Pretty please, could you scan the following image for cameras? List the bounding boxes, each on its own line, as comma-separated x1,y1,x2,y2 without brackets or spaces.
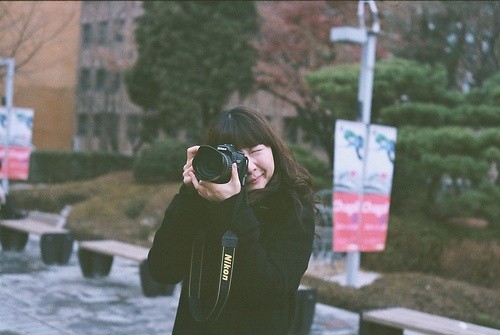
192,143,248,188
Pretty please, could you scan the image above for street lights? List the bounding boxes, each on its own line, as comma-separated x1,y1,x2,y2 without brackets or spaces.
331,1,380,287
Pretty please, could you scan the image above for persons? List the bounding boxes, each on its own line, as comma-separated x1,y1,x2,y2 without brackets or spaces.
142,110,319,334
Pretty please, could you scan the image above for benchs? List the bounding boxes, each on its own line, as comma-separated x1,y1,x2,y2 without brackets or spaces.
357,306,500,335
0,219,74,264
77,240,177,298
293,282,317,335
